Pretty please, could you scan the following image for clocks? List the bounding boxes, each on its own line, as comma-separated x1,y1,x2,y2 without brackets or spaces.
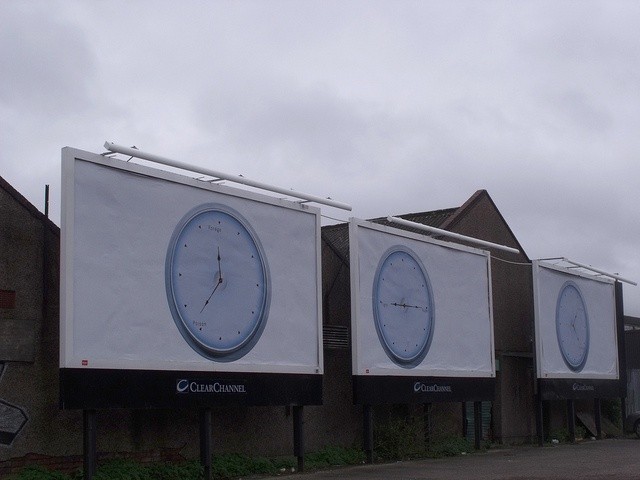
555,280,591,374
164,202,273,363
372,245,436,369
